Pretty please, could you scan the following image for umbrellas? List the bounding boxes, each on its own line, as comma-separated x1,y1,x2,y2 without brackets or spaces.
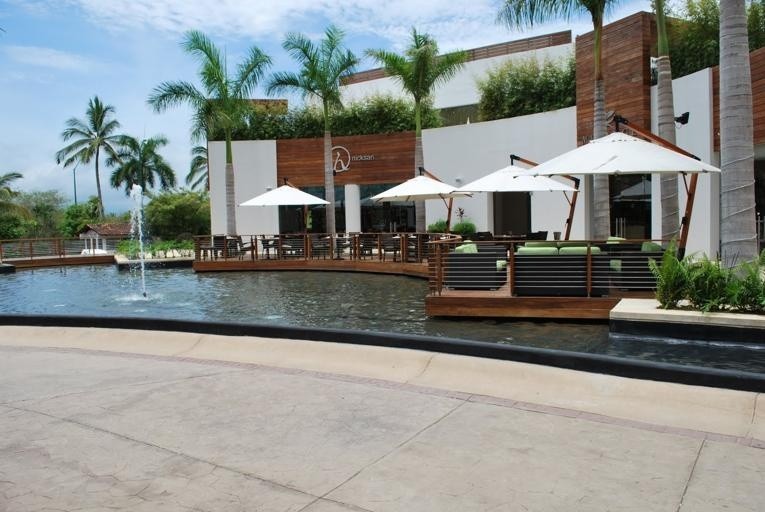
369,167,473,233
237,177,332,234
451,155,580,242
514,112,722,301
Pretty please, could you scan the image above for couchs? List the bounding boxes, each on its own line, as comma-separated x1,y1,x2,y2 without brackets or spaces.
429,225,669,299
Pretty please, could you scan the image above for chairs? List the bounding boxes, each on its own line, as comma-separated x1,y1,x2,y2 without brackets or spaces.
198,233,430,264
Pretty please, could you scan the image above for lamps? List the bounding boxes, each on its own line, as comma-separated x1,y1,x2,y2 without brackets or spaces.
674,110,690,125
